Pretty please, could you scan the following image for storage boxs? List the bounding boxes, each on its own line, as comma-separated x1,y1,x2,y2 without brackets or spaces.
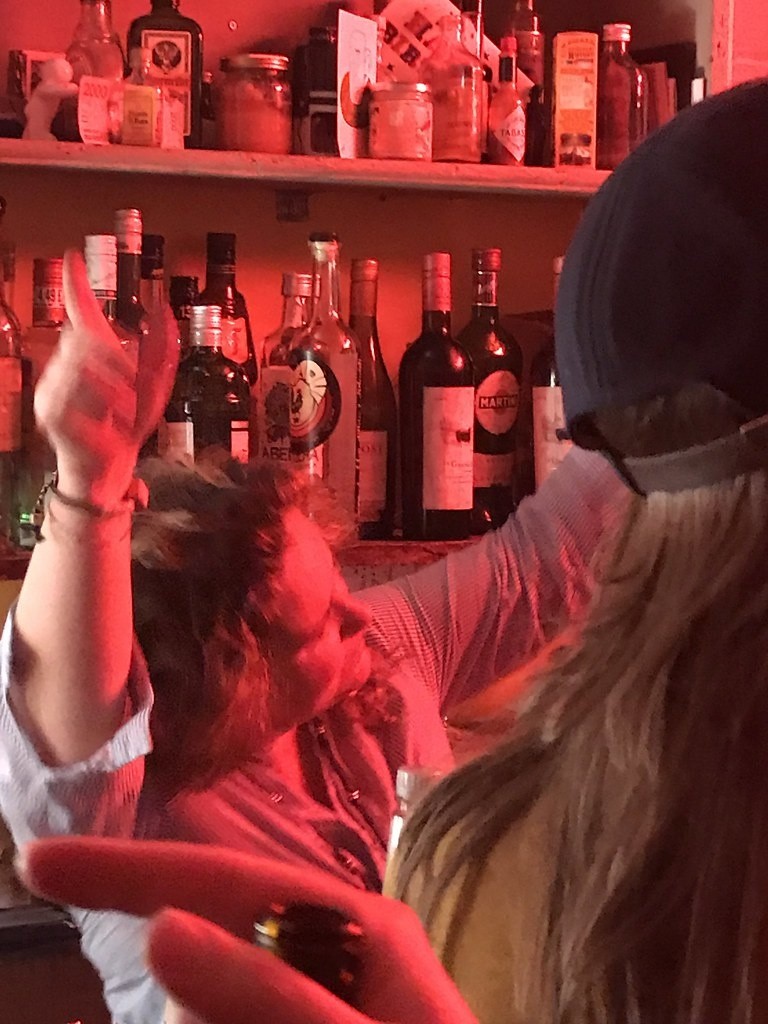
554,31,598,167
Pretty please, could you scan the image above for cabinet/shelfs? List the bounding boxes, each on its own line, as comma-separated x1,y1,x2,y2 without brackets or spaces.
0,1,734,588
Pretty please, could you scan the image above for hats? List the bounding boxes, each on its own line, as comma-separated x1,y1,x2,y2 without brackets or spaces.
554,75,768,493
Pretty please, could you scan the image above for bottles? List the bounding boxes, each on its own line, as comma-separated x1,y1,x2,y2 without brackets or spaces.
490,0,545,166
533,255,576,494
285,233,347,508
63,0,132,145
597,23,650,170
251,900,365,1010
204,26,336,156
167,232,258,468
261,273,330,483
121,47,163,145
381,764,433,893
454,248,524,536
125,0,203,147
82,208,165,459
398,250,474,540
0,242,70,548
422,0,492,163
348,257,397,541
368,82,432,160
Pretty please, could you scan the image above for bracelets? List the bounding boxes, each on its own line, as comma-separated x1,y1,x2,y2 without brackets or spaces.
19,477,149,543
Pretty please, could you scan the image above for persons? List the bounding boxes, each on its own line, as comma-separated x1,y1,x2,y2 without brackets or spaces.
16,837,475,1024
382,76,768,1024
0,246,626,1024
